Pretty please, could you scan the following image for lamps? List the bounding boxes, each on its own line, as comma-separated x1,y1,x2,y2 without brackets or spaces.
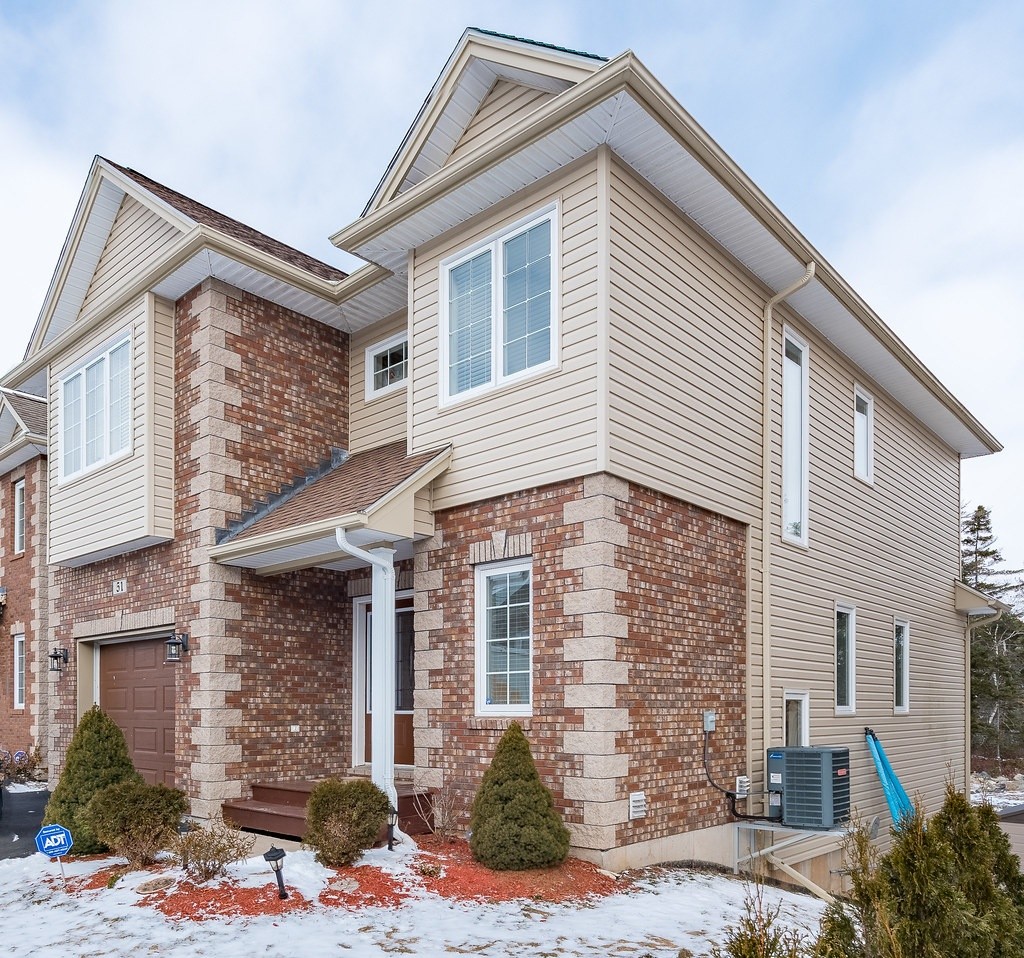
263,842,289,900
178,818,203,870
164,632,189,662
48,647,68,672
386,805,399,851
